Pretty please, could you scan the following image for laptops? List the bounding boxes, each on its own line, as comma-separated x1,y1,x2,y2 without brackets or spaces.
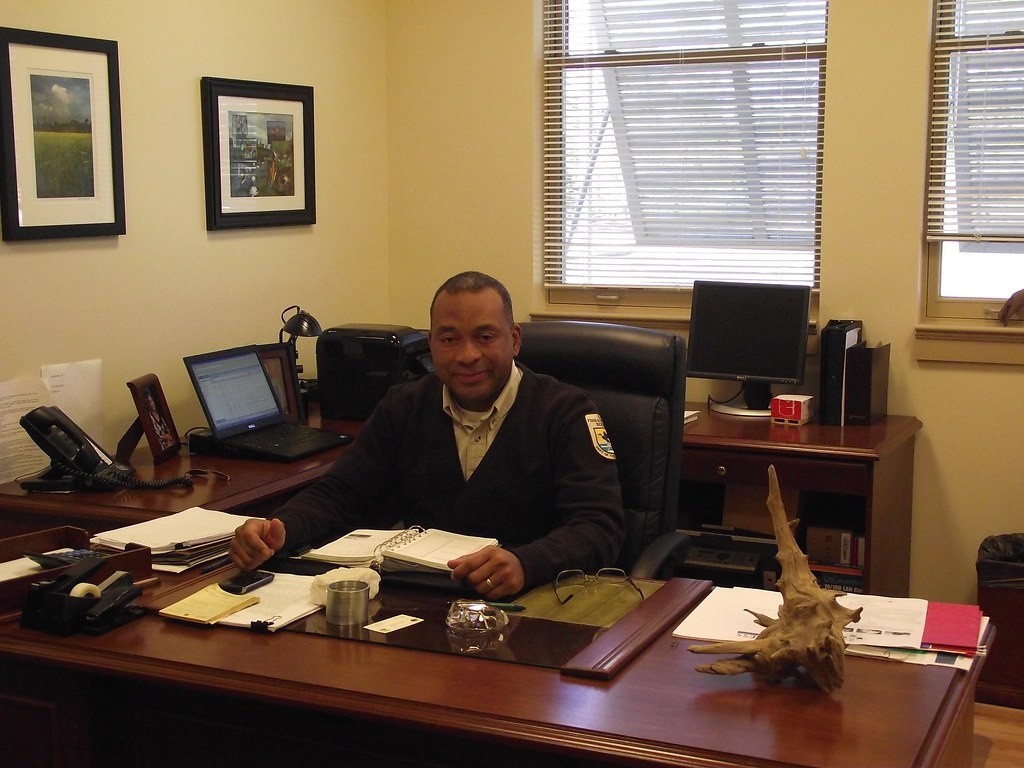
183,345,355,459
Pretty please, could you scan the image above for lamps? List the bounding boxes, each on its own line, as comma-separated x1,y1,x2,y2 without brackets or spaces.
279,305,322,414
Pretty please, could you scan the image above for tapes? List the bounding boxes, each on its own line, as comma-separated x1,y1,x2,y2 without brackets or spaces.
70,570,128,597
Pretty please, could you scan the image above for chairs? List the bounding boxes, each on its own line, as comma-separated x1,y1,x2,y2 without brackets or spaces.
511,319,692,583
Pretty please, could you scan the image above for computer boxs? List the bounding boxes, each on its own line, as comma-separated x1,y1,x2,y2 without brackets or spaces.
818,319,862,426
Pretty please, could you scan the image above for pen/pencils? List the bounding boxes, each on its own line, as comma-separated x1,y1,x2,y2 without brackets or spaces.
200,557,231,574
447,600,526,611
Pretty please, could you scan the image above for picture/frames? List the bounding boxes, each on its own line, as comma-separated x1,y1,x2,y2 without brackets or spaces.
111,374,182,463
201,76,318,231
259,344,307,424
0,26,127,241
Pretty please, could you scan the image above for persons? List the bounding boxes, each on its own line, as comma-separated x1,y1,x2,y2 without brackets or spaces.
147,395,175,449
228,272,626,601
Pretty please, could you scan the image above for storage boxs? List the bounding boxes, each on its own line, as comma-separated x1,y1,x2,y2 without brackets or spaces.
805,526,857,567
722,483,800,542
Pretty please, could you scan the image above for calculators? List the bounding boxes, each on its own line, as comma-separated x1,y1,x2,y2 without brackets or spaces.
22,549,111,569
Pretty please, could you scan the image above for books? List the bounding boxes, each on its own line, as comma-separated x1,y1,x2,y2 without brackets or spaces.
89,506,266,573
159,569,326,632
302,526,502,572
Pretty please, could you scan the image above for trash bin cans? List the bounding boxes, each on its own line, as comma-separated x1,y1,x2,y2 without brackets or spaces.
976,533,1024,709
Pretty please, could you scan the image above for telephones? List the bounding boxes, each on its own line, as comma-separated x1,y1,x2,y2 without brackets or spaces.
19,405,135,490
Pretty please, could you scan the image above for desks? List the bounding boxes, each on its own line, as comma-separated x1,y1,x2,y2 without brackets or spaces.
0,403,995,768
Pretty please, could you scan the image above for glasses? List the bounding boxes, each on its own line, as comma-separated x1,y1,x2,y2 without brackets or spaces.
553,568,644,606
184,468,231,483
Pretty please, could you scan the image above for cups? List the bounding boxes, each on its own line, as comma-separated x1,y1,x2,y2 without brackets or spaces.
326,580,369,626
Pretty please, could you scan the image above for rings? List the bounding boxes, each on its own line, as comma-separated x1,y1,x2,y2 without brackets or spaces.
486,578,494,589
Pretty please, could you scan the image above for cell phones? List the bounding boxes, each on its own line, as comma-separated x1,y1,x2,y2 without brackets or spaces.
218,571,275,596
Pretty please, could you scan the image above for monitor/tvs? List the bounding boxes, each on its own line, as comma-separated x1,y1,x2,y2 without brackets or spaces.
686,280,811,416
